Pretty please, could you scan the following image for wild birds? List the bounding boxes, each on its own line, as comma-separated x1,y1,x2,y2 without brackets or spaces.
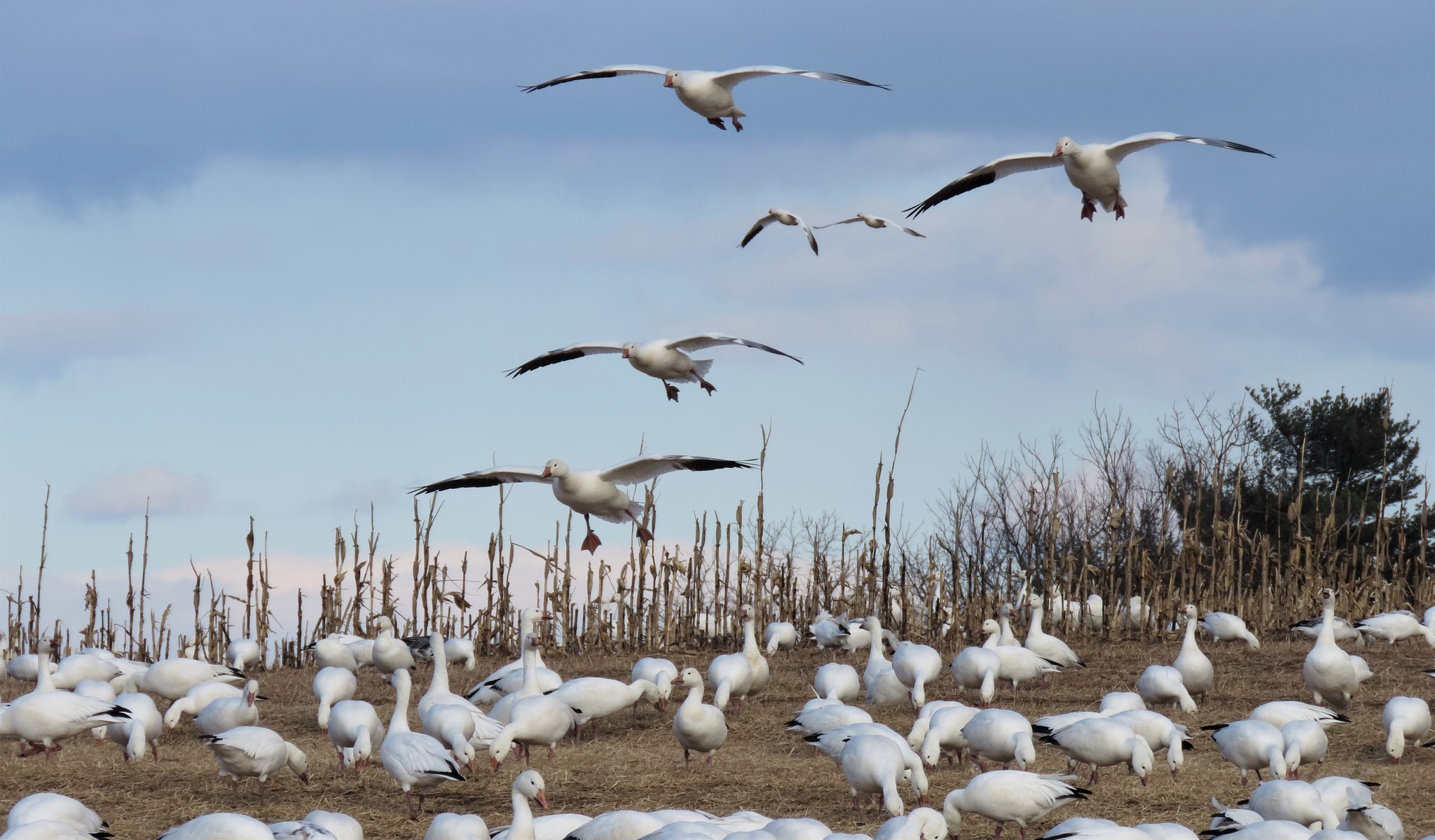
516,65,892,133
900,132,1276,223
405,455,762,556
736,208,818,258
813,213,927,239
502,333,805,403
0,569,1435,840
